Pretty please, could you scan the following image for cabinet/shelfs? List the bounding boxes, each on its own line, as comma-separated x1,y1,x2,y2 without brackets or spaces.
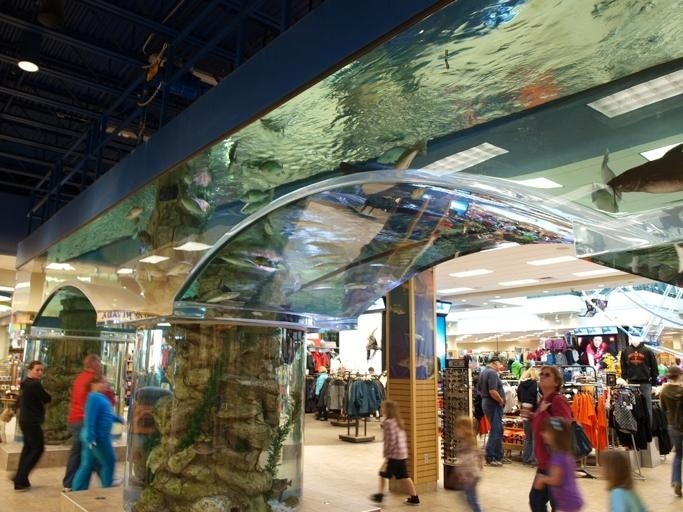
605,384,667,480
499,363,599,468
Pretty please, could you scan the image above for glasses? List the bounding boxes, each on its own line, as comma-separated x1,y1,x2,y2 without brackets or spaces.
539,373,550,377
441,369,468,462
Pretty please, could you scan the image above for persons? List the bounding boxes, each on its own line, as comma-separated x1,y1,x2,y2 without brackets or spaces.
529,366,573,512
581,336,608,374
72,376,128,491
598,451,645,512
621,333,659,434
452,417,482,511
62,354,116,492
371,401,420,506
478,357,511,467
662,367,683,498
369,367,374,375
134,365,174,391
517,368,542,466
533,417,584,511
675,358,682,372
15,361,51,491
315,366,328,421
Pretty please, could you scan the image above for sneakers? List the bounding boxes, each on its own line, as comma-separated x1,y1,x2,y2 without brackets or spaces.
405,496,419,505
489,461,502,467
501,458,512,463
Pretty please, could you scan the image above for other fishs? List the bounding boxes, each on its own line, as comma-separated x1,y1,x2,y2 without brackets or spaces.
122,69,473,323
605,143,681,194
599,147,623,200
590,182,618,213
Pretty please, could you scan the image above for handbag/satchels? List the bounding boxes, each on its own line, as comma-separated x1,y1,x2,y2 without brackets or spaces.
573,421,592,458
